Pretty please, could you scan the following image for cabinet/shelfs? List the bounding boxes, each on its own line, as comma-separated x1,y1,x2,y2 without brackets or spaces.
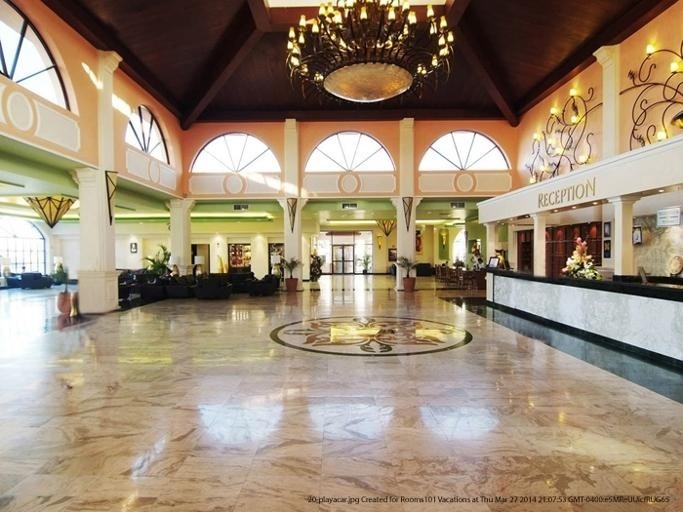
226,241,251,273
515,221,603,278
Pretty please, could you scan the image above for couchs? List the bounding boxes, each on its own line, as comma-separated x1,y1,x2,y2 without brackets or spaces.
389,264,396,276
413,262,431,278
112,263,280,309
4,256,67,291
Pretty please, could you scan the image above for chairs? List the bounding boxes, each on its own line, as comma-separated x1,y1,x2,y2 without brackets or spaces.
432,261,488,292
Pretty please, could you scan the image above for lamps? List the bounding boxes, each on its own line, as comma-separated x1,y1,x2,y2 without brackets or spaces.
286,197,297,235
105,168,117,228
442,233,446,250
168,255,180,278
402,196,414,231
376,219,396,238
522,31,682,187
272,0,459,108
23,195,77,232
376,235,381,250
269,256,280,276
195,255,204,275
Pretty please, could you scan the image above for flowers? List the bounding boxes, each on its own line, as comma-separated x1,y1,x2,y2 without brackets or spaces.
559,235,602,280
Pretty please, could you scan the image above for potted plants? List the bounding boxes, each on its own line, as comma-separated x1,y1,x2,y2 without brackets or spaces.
280,257,303,292
357,253,370,275
47,261,72,316
395,252,422,293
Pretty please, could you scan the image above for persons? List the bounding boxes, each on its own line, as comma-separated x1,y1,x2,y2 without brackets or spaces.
471,249,485,271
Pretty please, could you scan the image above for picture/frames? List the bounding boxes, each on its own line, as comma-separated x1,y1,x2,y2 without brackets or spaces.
487,257,499,269
387,248,397,262
129,242,136,253
603,221,612,239
603,238,611,258
